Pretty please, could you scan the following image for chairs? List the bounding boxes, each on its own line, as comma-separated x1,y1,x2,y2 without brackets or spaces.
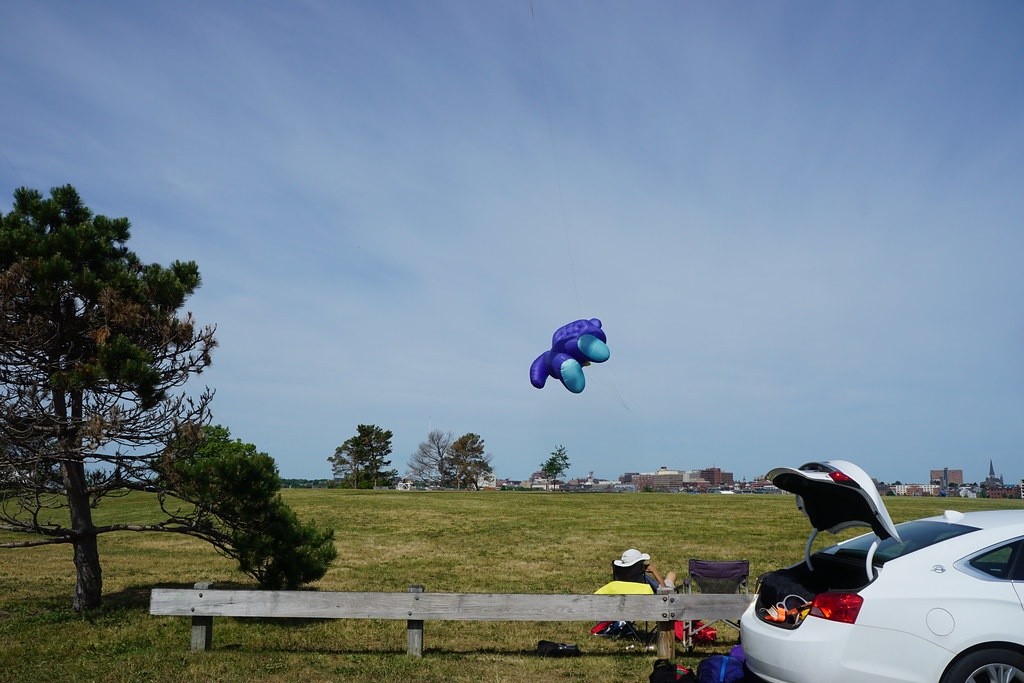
612,559,682,647
682,558,750,653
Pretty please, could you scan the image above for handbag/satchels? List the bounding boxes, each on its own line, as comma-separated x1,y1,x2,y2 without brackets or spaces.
650,657,697,683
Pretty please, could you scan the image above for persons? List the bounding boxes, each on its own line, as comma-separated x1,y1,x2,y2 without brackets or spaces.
613,548,677,589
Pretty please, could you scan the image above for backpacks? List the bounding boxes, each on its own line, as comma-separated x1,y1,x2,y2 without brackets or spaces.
697,653,746,683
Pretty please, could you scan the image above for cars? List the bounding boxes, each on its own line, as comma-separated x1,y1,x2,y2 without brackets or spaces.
740,460,1024,683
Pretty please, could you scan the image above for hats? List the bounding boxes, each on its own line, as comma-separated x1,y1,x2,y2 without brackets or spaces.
613,549,651,568
